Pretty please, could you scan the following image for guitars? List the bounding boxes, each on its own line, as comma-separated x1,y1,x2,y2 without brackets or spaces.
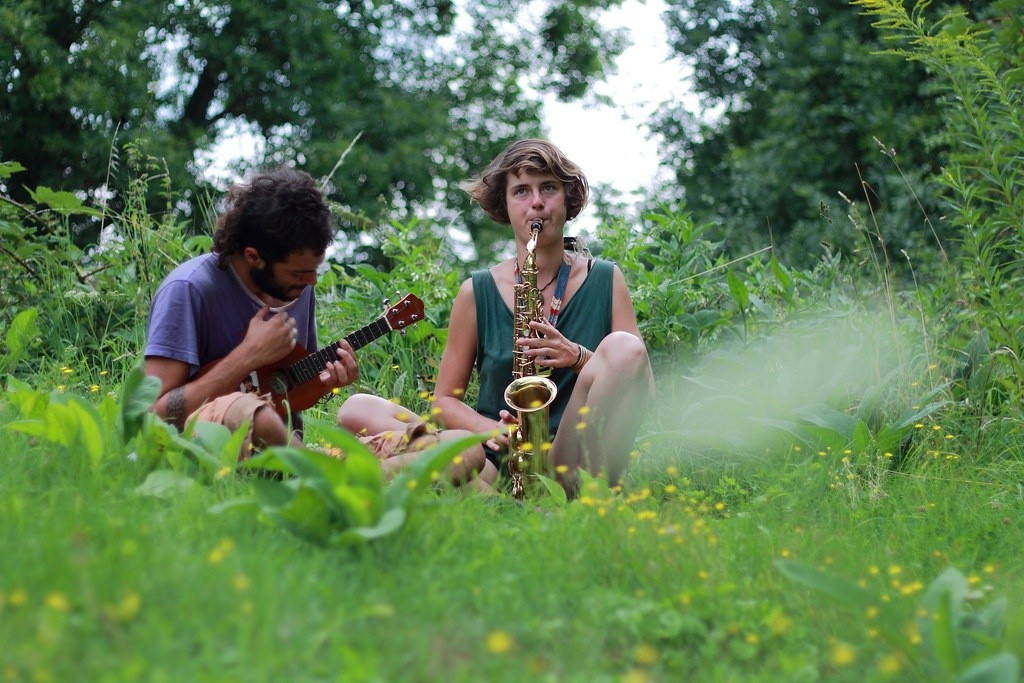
195,291,424,419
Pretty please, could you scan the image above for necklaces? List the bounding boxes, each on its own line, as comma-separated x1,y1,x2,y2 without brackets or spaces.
518,266,558,292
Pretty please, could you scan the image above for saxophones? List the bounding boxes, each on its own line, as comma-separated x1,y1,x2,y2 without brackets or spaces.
504,215,557,509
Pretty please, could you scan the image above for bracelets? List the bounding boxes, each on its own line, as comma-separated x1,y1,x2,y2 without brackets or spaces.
572,343,587,371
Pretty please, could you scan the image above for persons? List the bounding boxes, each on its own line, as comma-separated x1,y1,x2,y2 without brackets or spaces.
335,138,657,501
143,171,361,472
